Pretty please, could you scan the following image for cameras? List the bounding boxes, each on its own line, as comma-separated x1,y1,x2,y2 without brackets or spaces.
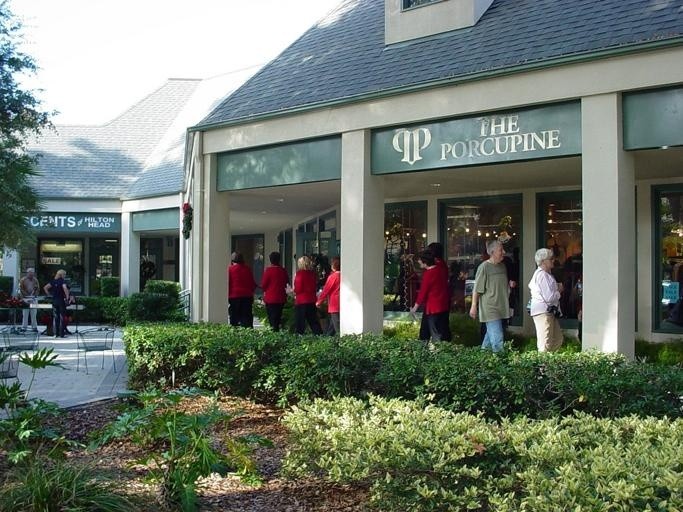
549,307,561,317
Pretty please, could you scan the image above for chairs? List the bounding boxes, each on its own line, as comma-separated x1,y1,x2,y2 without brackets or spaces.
76,326,116,375
0,326,40,375
0,348,21,388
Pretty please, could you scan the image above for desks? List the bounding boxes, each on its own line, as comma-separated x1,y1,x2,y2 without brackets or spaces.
1,302,87,338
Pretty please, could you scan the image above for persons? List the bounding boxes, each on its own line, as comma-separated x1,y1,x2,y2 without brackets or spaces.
259,249,288,332
469,240,516,354
419,242,449,341
228,249,257,329
285,254,322,333
528,248,565,353
410,249,451,343
18,267,74,338
315,255,341,335
